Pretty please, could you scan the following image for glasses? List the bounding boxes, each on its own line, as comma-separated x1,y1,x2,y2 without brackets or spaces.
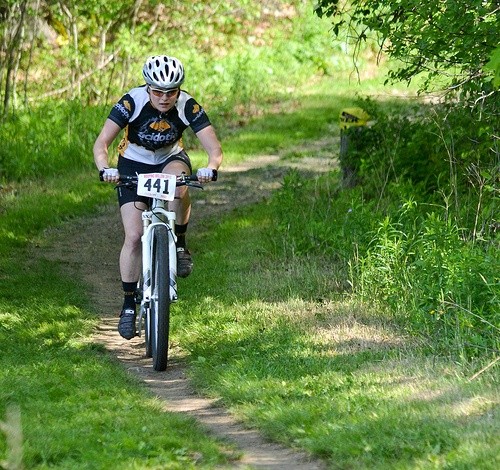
148,86,179,97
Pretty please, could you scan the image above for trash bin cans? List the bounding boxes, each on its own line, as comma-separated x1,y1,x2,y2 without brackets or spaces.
340,107,367,180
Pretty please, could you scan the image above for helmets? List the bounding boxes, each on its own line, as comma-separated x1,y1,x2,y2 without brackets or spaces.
142,55,185,88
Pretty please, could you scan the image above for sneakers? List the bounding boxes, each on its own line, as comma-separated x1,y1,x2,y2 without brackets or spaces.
118,308,137,340
176,247,193,278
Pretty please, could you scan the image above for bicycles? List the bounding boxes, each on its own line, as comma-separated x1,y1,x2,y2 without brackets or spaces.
99,166,218,370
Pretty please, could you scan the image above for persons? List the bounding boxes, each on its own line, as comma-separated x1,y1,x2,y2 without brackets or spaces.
92,54,224,339
